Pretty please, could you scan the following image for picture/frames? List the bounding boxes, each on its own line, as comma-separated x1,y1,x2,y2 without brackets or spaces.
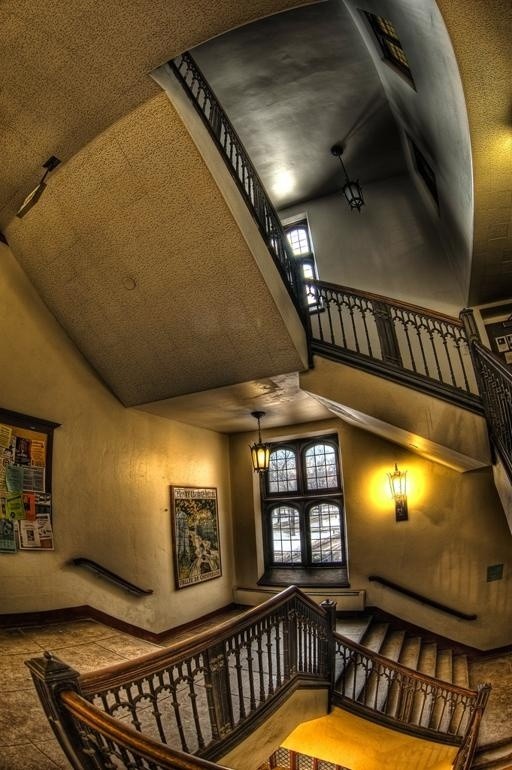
168,486,222,591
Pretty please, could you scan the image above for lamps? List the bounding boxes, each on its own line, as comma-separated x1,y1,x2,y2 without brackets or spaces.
386,463,409,521
330,146,365,213
248,411,271,473
